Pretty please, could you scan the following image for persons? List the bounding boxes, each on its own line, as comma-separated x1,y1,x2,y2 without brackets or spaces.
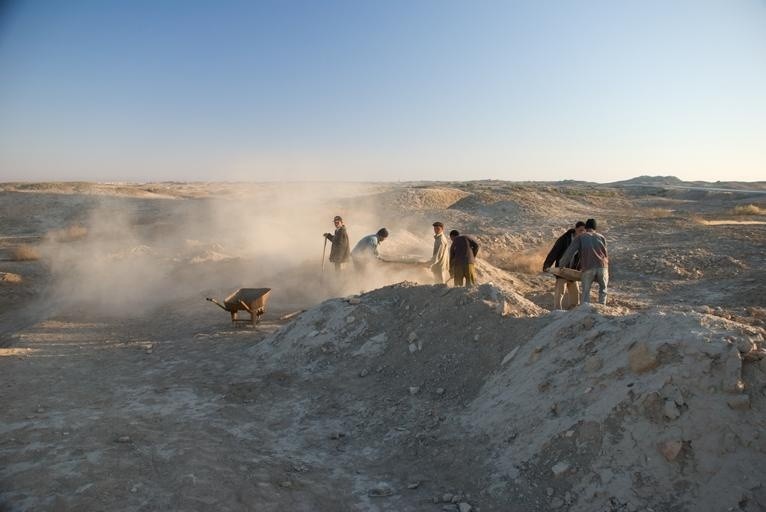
350,227,389,270
449,229,479,287
543,221,586,310
323,216,350,269
424,221,449,284
559,219,609,306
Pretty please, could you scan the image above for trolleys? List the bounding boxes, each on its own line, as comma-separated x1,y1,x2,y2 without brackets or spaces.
206,287,271,327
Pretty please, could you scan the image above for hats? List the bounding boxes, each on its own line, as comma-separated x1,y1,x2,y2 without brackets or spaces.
333,215,343,222
